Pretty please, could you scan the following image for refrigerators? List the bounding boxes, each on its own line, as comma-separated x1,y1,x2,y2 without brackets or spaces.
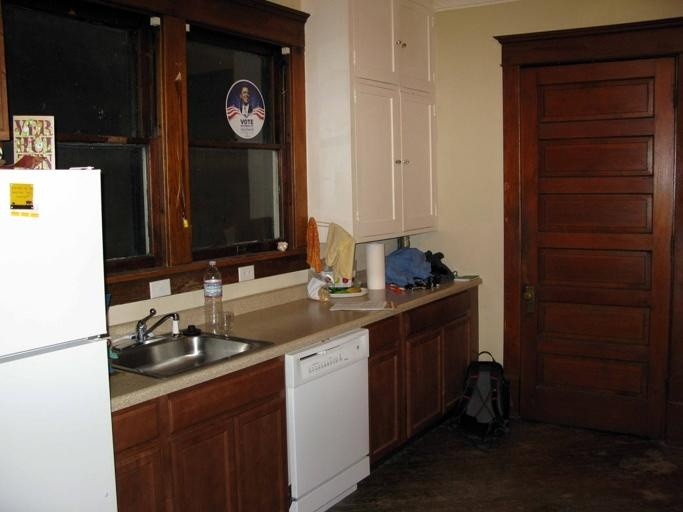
0,169,118,511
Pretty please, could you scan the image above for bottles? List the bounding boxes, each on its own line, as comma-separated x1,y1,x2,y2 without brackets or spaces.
203,262,224,335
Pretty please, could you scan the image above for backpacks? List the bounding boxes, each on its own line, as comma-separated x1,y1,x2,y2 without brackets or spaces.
457,352,511,450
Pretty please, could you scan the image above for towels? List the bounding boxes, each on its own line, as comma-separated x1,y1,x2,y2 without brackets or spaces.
325,223,356,279
307,217,321,273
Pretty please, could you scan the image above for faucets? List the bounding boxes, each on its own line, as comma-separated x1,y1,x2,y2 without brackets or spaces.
134,307,179,343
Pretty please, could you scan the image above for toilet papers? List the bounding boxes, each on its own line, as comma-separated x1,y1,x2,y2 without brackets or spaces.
365,242,386,290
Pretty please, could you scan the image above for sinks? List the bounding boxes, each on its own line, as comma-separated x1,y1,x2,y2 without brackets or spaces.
110,330,275,380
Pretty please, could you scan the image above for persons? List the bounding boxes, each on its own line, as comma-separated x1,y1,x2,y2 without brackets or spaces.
239,86,252,117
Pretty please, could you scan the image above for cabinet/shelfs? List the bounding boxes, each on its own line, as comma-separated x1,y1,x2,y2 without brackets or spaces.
111,385,168,512
404,279,481,441
306,83,439,243
301,0,434,95
162,345,287,512
363,307,404,464
